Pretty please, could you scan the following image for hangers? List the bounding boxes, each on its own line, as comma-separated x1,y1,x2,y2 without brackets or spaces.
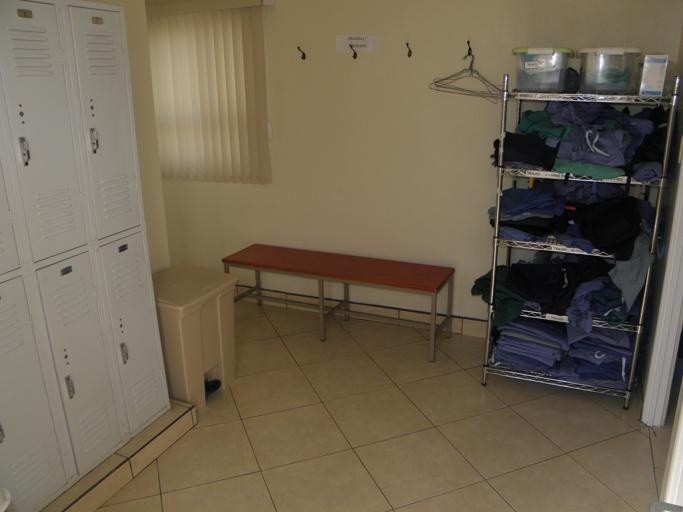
427,53,504,104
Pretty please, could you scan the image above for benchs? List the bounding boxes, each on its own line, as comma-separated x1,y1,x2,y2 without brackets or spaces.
221,243,456,363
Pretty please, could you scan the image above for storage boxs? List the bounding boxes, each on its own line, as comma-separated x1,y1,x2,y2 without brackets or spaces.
512,46,642,94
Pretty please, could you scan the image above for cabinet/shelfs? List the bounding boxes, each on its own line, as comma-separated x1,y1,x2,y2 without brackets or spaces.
480,74,682,410
0,1,172,512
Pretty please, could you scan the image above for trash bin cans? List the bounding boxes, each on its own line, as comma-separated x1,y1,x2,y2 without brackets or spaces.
152,263,240,406
